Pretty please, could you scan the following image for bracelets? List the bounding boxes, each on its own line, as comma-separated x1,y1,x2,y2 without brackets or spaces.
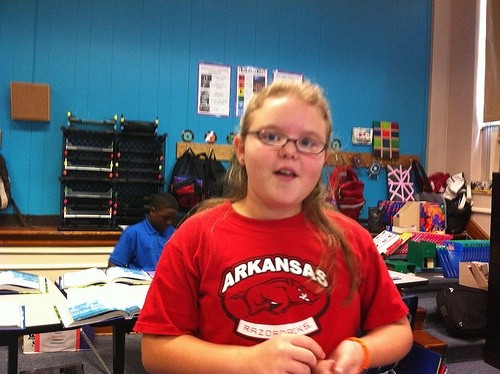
347,336,369,371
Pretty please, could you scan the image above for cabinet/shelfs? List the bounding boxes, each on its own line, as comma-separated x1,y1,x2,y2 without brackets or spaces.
57,111,168,230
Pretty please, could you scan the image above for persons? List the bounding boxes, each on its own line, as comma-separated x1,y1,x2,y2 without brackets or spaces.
132,76,415,373
106,190,179,273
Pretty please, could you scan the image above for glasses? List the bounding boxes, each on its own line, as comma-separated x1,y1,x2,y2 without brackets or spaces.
246,128,328,156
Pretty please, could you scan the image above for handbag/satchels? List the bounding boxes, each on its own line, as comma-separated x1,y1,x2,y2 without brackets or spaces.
442,173,476,234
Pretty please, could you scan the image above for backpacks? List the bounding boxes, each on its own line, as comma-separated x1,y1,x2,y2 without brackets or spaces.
326,154,367,223
168,147,227,214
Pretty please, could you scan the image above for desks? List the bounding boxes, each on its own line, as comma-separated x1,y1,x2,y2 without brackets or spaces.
0,271,458,373
0,216,146,271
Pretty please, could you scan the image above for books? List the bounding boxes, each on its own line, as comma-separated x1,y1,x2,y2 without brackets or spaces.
373,198,490,290
0,265,153,331
389,340,449,374
